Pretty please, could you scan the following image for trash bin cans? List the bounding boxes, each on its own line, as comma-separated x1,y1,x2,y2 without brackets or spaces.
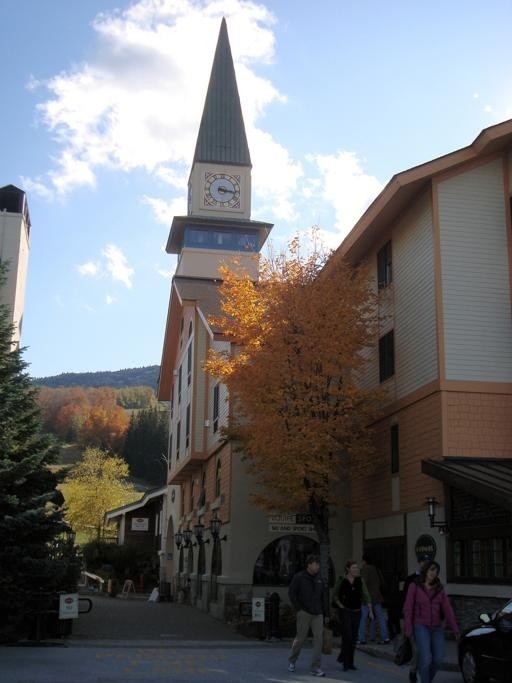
158,582,171,603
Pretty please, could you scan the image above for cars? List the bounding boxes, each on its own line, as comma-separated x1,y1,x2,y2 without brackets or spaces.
457,598,512,683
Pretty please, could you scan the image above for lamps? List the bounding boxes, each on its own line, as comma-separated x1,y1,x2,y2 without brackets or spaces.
419,497,447,536
174,514,227,550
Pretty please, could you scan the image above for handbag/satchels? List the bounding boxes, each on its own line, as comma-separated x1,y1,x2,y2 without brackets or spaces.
321,623,335,655
394,637,414,667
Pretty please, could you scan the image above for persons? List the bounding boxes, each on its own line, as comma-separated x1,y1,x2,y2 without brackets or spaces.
86,558,160,593
356,552,390,645
332,559,375,671
401,551,447,682
287,553,330,676
404,560,461,682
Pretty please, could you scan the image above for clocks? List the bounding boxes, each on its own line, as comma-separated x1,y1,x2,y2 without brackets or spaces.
200,171,243,214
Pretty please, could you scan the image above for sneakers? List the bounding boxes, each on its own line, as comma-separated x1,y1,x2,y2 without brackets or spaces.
342,663,358,673
310,668,327,677
287,659,297,672
408,670,417,683
